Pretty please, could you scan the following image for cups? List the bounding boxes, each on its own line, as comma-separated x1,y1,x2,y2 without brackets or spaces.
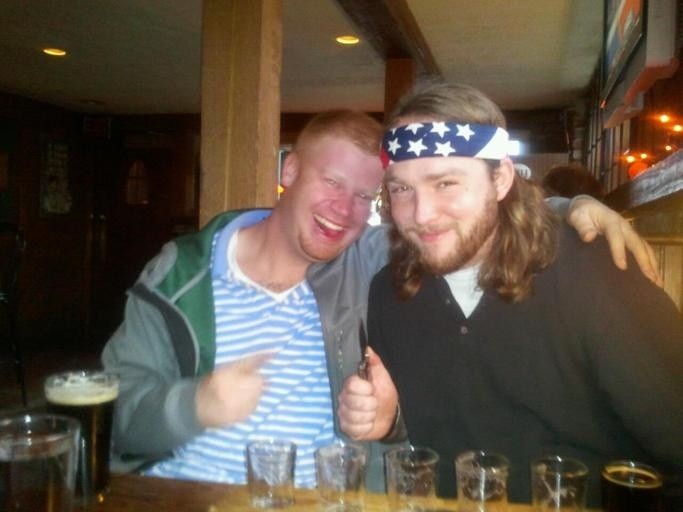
315,444,367,512
0,413,82,512
247,440,297,508
529,455,589,511
453,450,510,511
40,372,121,506
600,459,662,511
384,446,440,512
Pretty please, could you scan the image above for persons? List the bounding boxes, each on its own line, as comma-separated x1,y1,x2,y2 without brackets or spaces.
97,103,664,490
539,161,604,206
334,67,682,512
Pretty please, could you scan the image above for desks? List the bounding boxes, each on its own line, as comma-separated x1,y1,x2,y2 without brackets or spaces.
92,472,599,512
